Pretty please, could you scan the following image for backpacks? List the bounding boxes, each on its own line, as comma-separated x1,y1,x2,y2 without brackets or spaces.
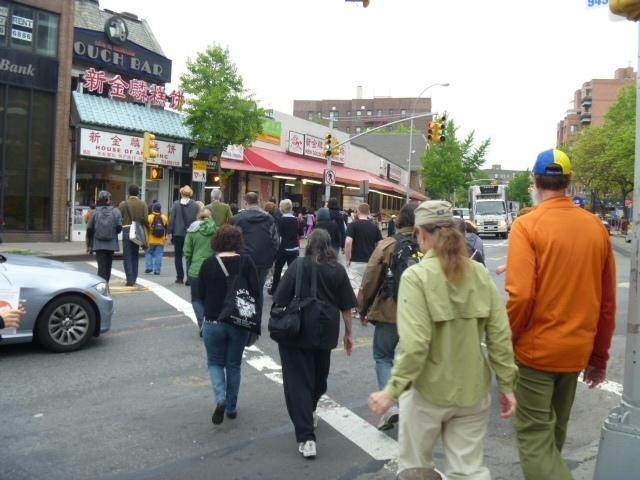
94,207,115,242
385,233,423,301
151,214,165,238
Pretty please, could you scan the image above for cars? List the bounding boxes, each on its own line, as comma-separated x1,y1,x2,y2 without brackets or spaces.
0,250,116,356
452,206,520,231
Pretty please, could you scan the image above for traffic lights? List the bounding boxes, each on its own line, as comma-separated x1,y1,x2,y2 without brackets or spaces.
333,136,340,156
324,133,332,159
436,115,446,145
426,120,436,143
150,166,164,180
142,132,157,161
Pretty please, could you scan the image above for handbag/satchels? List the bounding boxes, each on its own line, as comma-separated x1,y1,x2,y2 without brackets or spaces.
129,221,146,246
268,298,340,351
218,276,262,336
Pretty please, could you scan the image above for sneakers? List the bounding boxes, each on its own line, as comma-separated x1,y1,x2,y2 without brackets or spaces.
126,282,133,286
145,269,159,275
267,288,273,297
313,412,319,428
299,440,316,458
228,412,236,418
175,279,190,285
378,407,398,430
351,307,367,319
213,402,226,426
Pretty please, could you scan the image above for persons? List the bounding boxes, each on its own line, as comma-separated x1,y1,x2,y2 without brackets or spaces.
199,224,262,424
366,200,521,480
463,220,478,235
506,147,617,480
87,185,148,285
164,184,201,285
303,197,383,294
494,264,506,275
272,226,359,458
233,191,300,296
452,215,485,267
182,207,219,336
388,213,398,236
204,189,233,223
0,306,27,342
355,203,423,431
144,203,166,275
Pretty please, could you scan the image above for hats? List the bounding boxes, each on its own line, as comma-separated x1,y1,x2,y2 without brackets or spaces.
153,202,161,212
180,185,193,197
532,148,571,176
316,207,330,220
412,200,455,238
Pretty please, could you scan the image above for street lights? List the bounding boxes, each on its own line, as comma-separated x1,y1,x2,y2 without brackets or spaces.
405,82,451,206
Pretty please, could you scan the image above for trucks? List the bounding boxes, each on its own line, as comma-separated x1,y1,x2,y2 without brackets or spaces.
507,200,520,215
467,183,511,240
